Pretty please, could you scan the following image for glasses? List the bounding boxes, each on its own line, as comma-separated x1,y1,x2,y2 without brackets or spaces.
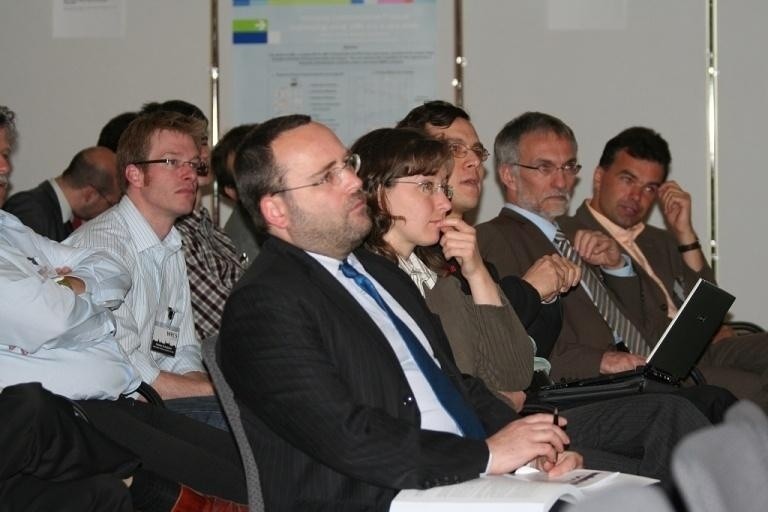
268,151,362,197
445,141,490,162
504,155,583,177
381,178,455,200
124,157,202,172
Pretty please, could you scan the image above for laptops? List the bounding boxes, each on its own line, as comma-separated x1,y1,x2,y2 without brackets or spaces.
537,276,736,401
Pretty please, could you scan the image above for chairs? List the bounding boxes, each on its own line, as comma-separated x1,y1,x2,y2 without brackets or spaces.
198,333,265,512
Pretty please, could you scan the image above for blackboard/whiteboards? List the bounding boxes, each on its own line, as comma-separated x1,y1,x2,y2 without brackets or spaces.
0,3,717,297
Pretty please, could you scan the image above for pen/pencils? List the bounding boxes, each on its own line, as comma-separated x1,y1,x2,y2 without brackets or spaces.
553,408,559,426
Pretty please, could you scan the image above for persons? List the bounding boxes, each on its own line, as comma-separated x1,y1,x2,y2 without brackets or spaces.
349,125,537,416
0,97,276,510
208,115,713,512
471,110,767,414
573,125,736,387
398,96,740,425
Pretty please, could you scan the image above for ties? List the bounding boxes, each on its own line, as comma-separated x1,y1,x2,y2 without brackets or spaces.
338,258,488,440
552,229,652,359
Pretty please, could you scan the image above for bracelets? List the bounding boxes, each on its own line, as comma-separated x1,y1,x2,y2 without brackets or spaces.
677,240,702,252
